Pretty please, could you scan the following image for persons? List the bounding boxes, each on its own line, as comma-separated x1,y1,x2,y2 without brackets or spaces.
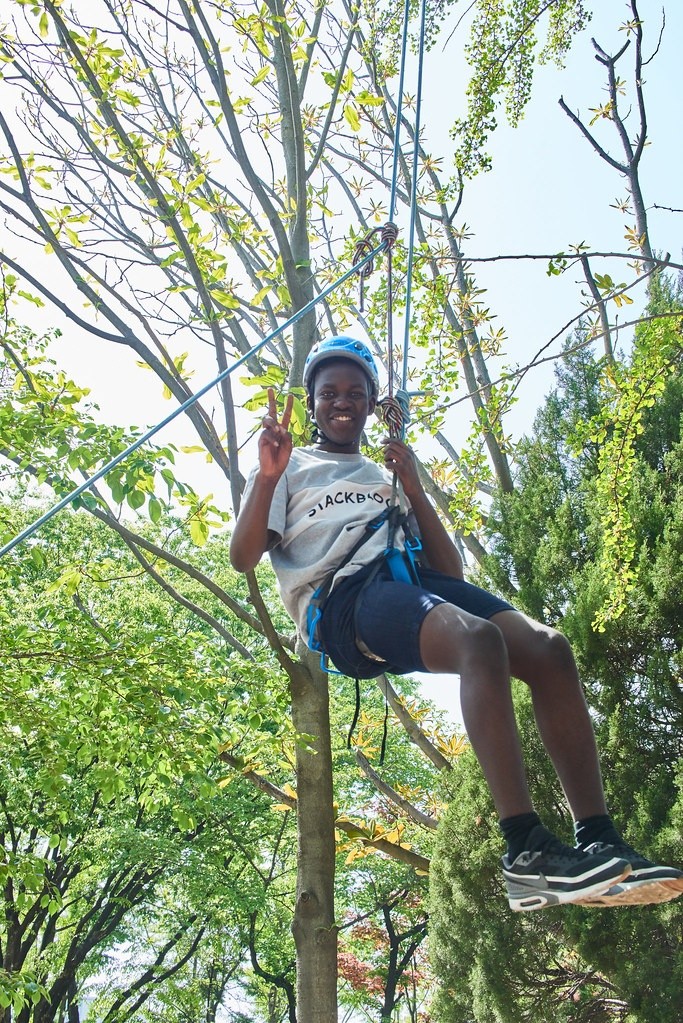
229,338,683,911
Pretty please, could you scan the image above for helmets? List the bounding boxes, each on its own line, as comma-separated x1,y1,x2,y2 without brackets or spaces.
301,336,380,402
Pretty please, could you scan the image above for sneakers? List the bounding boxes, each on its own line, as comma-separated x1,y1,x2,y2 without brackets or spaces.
500,823,631,912
573,827,683,907
